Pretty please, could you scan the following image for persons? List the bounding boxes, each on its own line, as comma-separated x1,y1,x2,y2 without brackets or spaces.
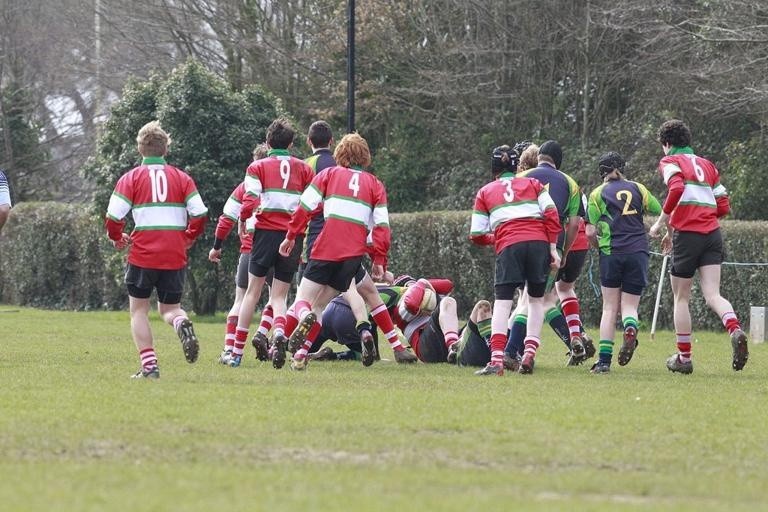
649,119,749,374
390,138,595,375
105,121,209,379
584,152,674,372
0,170,12,228
209,118,420,371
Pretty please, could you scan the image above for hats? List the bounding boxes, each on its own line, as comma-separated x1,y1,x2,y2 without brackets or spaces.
491,140,562,173
599,151,625,178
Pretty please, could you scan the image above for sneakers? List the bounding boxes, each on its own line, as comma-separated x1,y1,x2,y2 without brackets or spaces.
360,329,377,367
447,335,463,362
178,318,199,363
731,329,748,371
218,311,333,368
667,354,693,374
566,333,610,373
474,352,534,376
618,323,638,366
132,368,160,379
394,347,418,362
